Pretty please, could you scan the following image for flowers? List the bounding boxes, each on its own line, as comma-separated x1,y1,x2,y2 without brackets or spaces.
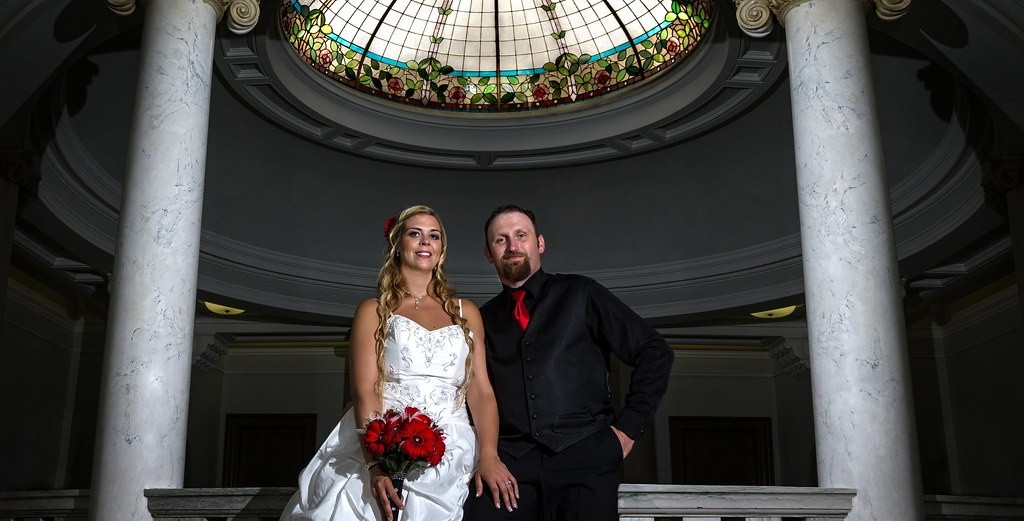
383,215,397,240
355,402,449,521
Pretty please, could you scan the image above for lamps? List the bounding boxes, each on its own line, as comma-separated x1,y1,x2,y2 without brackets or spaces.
749,305,798,319
204,301,245,315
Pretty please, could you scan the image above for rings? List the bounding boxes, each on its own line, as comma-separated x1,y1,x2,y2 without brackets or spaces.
506,481,511,485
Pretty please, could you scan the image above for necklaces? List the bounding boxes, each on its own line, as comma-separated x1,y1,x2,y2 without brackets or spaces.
405,290,426,310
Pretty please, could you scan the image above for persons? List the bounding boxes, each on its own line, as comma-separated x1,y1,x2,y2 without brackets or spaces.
462,203,675,521
279,205,519,521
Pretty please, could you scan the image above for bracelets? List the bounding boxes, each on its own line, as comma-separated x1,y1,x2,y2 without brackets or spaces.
366,460,380,471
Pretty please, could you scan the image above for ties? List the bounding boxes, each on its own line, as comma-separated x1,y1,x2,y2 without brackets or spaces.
510,289,531,331
